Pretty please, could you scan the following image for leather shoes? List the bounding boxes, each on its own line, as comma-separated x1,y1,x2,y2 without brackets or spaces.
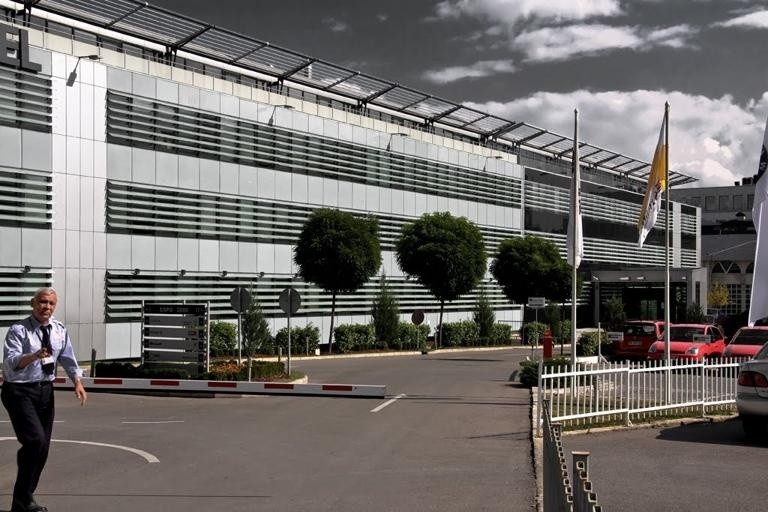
11,503,46,512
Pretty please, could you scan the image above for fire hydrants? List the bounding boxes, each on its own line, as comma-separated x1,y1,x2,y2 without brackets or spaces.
543,330,555,358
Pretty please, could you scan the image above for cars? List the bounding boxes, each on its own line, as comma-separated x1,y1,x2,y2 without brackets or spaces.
619,319,768,431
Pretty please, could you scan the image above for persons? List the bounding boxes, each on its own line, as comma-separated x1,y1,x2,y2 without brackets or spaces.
0,286,88,512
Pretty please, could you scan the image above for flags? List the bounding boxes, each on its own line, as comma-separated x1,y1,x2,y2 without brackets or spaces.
636,108,671,248
747,121,768,329
565,114,587,271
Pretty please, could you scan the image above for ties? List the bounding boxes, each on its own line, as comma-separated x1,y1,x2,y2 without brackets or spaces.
40,326,54,375
